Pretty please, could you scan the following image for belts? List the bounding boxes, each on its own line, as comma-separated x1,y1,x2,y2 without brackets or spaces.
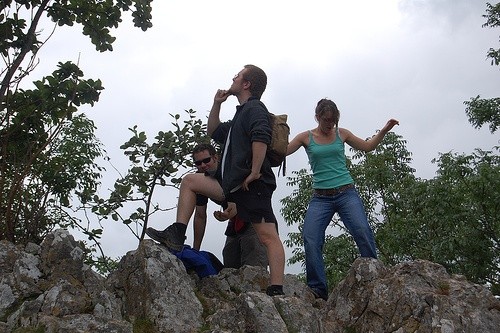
315,183,353,196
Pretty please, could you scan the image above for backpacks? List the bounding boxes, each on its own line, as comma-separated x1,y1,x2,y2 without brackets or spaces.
175,244,225,278
267,113,290,167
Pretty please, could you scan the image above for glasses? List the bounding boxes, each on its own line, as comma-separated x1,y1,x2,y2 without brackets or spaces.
194,154,211,166
234,74,248,81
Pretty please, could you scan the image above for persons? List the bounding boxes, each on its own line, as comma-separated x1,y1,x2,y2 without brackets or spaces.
284,99,399,301
193,143,278,270
144,65,286,296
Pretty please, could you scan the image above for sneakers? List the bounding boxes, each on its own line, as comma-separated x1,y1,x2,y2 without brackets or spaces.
266,285,285,296
145,222,187,251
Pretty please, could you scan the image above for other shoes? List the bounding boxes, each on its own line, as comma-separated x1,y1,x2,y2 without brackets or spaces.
306,286,328,301
